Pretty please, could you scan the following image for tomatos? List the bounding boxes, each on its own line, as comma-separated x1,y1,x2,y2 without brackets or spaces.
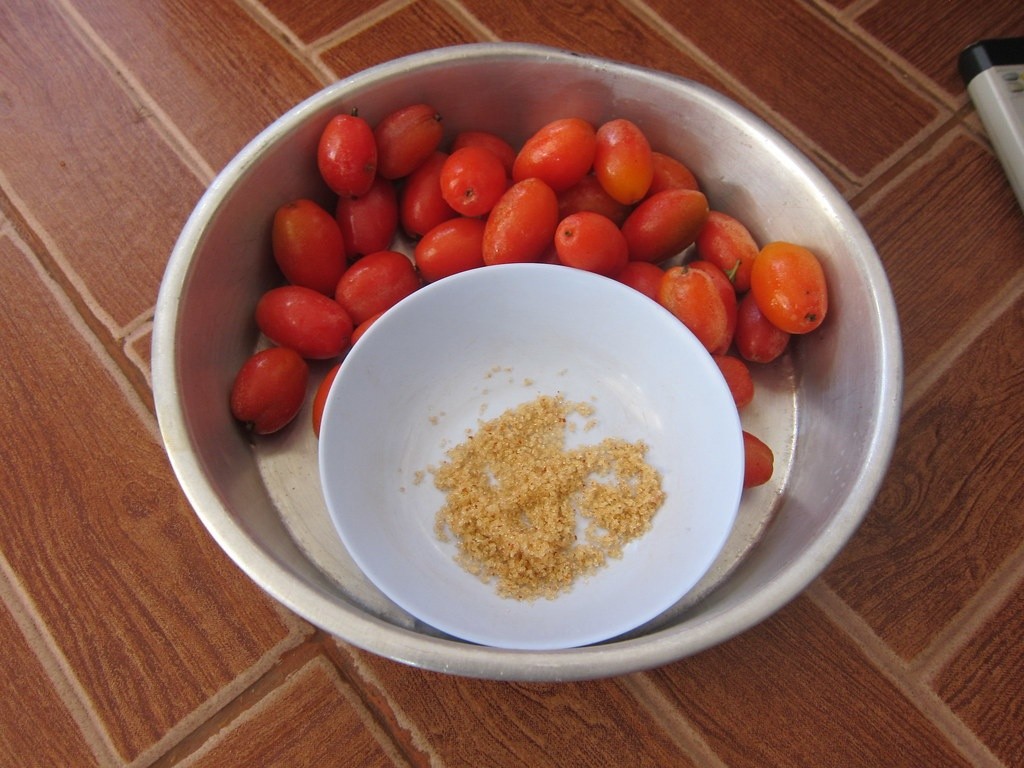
233,103,829,487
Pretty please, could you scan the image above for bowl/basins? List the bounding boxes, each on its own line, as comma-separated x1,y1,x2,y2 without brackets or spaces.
318,262,746,653
150,43,907,681
